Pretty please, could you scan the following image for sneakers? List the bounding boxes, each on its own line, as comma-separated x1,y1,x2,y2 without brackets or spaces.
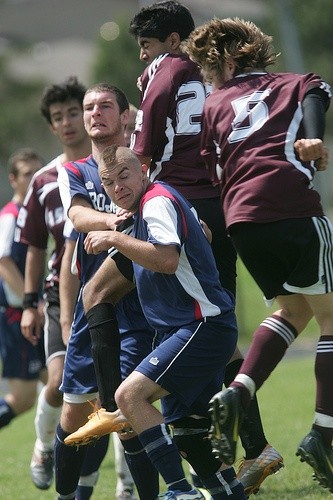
237,444,285,496
30,446,55,489
295,430,333,495
64,400,133,447
209,386,240,465
158,488,206,500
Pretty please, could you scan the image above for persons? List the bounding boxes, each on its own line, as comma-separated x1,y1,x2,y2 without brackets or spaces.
15,0,283,500
182,16,333,494
0,147,47,427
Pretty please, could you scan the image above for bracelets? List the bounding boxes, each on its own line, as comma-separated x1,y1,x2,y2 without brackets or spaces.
21,291,39,310
